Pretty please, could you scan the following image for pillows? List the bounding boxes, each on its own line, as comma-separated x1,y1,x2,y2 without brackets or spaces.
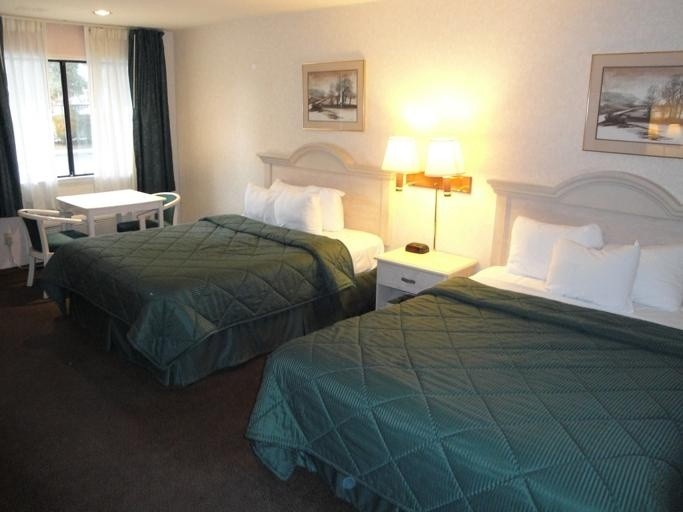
243,179,346,239
506,215,682,317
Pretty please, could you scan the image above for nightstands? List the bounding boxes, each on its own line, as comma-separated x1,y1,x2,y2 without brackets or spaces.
375,246,479,313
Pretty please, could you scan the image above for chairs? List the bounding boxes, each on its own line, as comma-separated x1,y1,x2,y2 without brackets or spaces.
17,188,181,301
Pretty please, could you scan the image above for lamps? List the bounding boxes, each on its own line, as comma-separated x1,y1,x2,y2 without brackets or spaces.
381,136,472,197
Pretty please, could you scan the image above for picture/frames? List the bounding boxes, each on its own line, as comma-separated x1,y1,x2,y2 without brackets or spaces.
301,59,365,133
582,50,683,160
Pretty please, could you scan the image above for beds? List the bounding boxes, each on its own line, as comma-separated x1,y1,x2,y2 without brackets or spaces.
271,172,683,511
57,142,392,388
